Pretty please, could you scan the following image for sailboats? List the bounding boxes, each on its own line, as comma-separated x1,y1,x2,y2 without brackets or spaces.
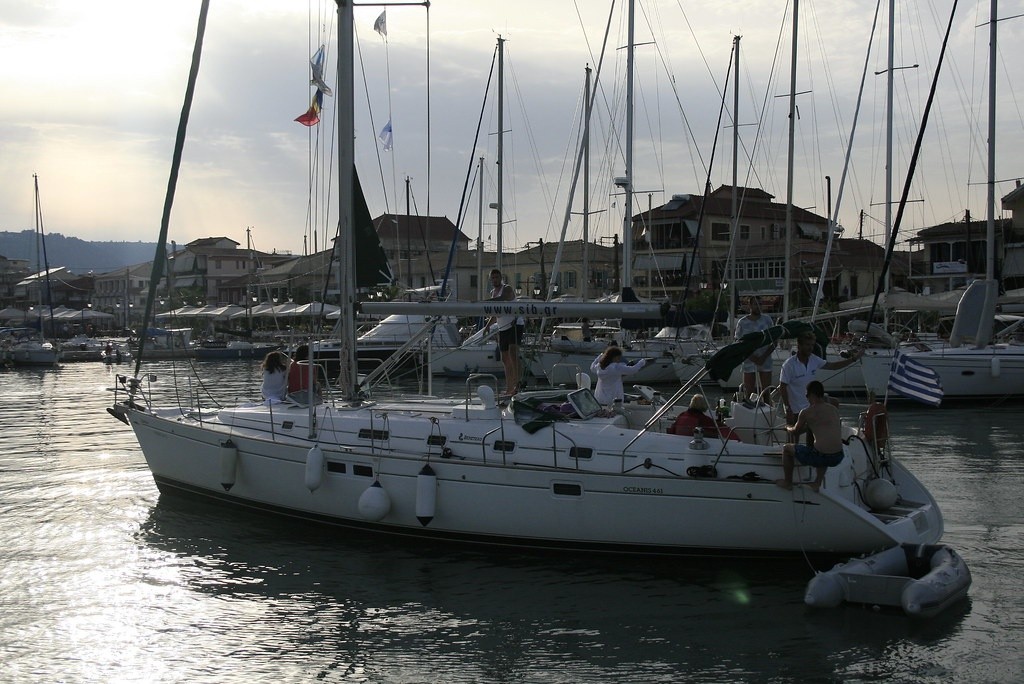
0,34,1024,412
95,1,966,562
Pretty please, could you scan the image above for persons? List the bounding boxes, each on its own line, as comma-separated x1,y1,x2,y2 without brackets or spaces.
589,347,649,410
734,295,779,405
580,318,595,343
260,345,323,405
671,393,729,438
843,286,849,299
483,270,525,397
774,331,866,491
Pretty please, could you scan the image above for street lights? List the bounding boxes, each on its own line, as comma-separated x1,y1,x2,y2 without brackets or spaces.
791,259,818,317
515,275,542,344
81,302,94,335
115,299,126,337
244,290,258,338
697,262,729,326
23,305,35,329
152,295,165,330
128,299,135,337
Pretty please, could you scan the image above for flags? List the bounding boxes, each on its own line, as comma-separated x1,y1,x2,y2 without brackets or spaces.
374,10,387,37
886,349,945,407
378,120,393,152
293,41,331,127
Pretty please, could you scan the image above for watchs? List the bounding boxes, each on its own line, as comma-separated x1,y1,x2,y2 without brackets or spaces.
852,355,857,362
785,405,790,410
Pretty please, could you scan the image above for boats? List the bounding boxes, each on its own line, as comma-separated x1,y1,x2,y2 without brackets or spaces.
799,542,973,622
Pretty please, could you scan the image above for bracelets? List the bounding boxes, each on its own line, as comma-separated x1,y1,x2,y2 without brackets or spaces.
750,355,758,362
786,411,791,413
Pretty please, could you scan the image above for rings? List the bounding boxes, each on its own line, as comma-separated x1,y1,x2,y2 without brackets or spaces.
646,362,647,364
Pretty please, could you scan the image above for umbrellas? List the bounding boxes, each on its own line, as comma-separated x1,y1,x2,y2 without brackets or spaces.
155,301,342,320
0,304,114,321
